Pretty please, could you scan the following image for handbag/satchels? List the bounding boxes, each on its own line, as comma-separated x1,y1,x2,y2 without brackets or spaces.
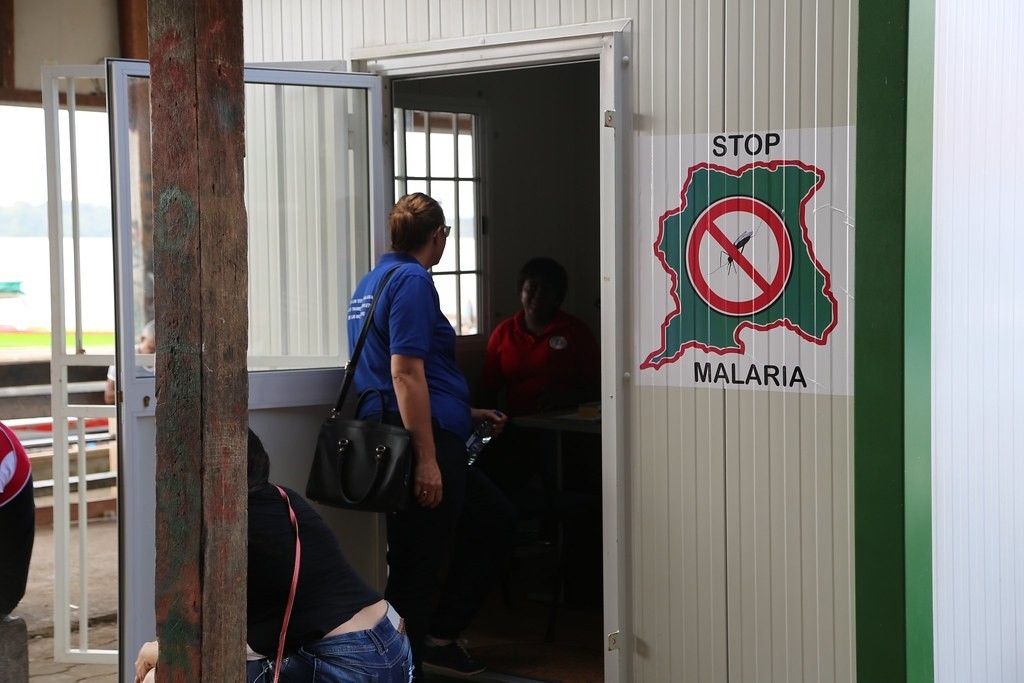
306,388,414,514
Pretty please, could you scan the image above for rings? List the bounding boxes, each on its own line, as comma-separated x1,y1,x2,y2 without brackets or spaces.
422,490,427,496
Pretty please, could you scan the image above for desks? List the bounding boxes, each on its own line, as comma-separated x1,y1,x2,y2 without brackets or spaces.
512,414,602,644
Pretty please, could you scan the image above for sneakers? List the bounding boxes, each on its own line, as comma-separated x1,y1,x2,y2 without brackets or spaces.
421,634,488,675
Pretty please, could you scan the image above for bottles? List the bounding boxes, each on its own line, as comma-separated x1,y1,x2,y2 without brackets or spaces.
464,411,502,466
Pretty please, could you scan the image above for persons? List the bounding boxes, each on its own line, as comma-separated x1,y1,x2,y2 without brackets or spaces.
133,426,415,683
105,319,155,404
346,192,518,683
0,421,37,622
480,257,601,417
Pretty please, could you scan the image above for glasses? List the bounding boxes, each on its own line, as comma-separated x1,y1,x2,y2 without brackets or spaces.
434,225,451,237
